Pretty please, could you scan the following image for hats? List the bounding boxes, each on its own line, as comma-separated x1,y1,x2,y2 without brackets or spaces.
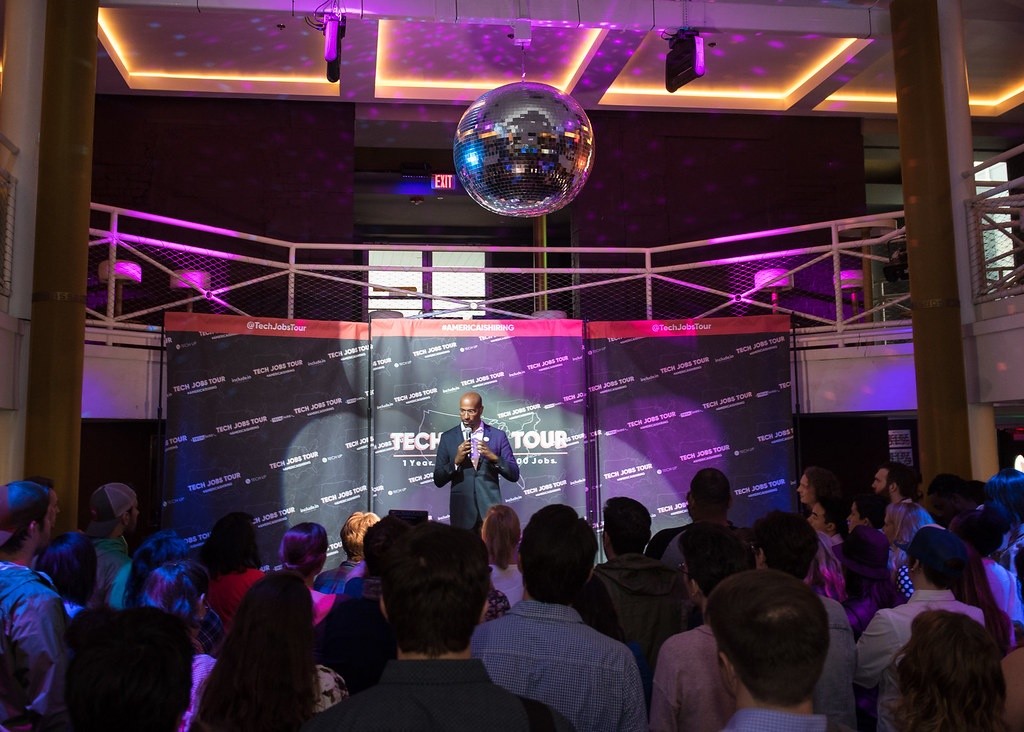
86,483,137,537
893,528,968,578
833,524,892,579
0,481,44,545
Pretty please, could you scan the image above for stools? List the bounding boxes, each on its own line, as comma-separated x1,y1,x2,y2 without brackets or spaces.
97,260,142,346
170,270,212,312
753,267,800,328
831,269,863,324
532,309,568,319
369,310,403,319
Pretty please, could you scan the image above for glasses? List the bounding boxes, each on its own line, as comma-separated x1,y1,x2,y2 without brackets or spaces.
749,540,759,555
675,561,689,575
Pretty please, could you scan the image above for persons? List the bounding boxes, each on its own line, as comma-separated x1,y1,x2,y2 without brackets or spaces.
434,392,520,533
0,463,1024,732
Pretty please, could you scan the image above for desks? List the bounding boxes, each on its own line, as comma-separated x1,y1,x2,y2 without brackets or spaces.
838,218,897,348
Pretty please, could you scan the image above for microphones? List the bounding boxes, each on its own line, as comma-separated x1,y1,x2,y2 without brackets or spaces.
464,426,473,459
